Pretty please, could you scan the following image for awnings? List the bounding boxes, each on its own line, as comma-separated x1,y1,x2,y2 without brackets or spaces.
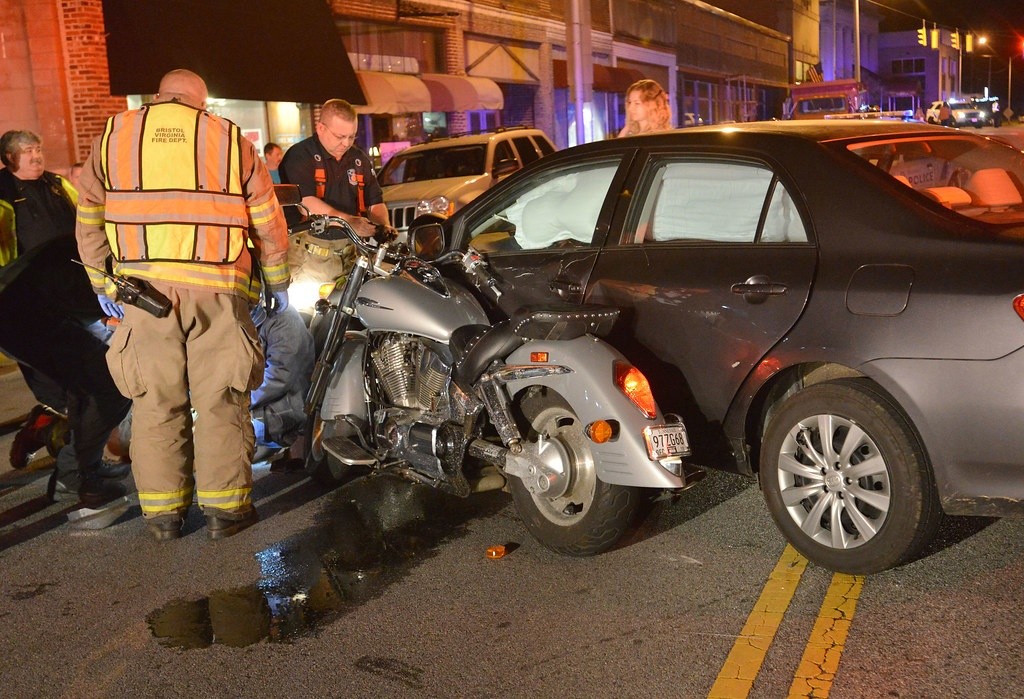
352,71,504,115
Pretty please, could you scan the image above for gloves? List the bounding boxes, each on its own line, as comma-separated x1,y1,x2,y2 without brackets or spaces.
98,294,124,318
265,288,288,318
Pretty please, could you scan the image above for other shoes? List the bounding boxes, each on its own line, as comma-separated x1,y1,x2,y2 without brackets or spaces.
145,504,258,539
9,404,53,469
48,447,129,508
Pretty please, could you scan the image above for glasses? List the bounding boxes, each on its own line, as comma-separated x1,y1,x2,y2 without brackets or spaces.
324,123,355,140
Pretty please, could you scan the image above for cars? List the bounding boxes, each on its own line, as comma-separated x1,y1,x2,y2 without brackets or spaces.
425,122,1024,576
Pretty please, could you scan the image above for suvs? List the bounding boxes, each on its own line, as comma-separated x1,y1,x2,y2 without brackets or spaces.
926,101,985,129
376,125,561,247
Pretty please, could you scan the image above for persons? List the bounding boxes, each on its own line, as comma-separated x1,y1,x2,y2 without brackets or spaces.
249,286,315,472
0,129,77,470
75,68,291,542
263,142,284,184
938,101,950,126
616,79,671,138
0,231,134,495
278,99,399,312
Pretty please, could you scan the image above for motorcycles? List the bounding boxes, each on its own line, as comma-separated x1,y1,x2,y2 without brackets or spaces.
271,181,706,556
